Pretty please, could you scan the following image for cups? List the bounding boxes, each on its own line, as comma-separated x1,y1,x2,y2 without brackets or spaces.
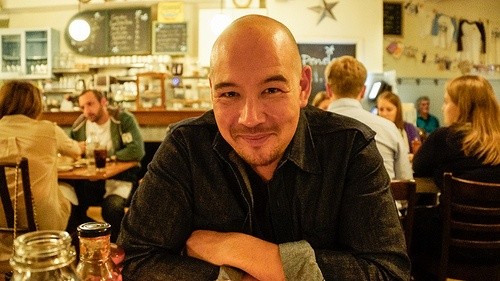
93,144,107,174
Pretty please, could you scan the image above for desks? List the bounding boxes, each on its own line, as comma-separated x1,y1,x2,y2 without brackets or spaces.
59,161,137,180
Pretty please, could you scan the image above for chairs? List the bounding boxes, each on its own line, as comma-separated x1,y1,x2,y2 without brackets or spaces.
0,157,37,238
391,172,500,281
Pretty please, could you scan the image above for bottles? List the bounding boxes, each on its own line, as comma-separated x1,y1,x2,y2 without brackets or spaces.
85,131,101,174
75,221,122,281
8,230,80,281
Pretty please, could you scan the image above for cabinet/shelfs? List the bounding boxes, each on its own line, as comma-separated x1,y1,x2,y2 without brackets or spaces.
0,27,213,109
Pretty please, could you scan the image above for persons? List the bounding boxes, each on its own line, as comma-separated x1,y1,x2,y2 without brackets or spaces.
0,80,145,265
116,14,412,281
312,56,500,281
